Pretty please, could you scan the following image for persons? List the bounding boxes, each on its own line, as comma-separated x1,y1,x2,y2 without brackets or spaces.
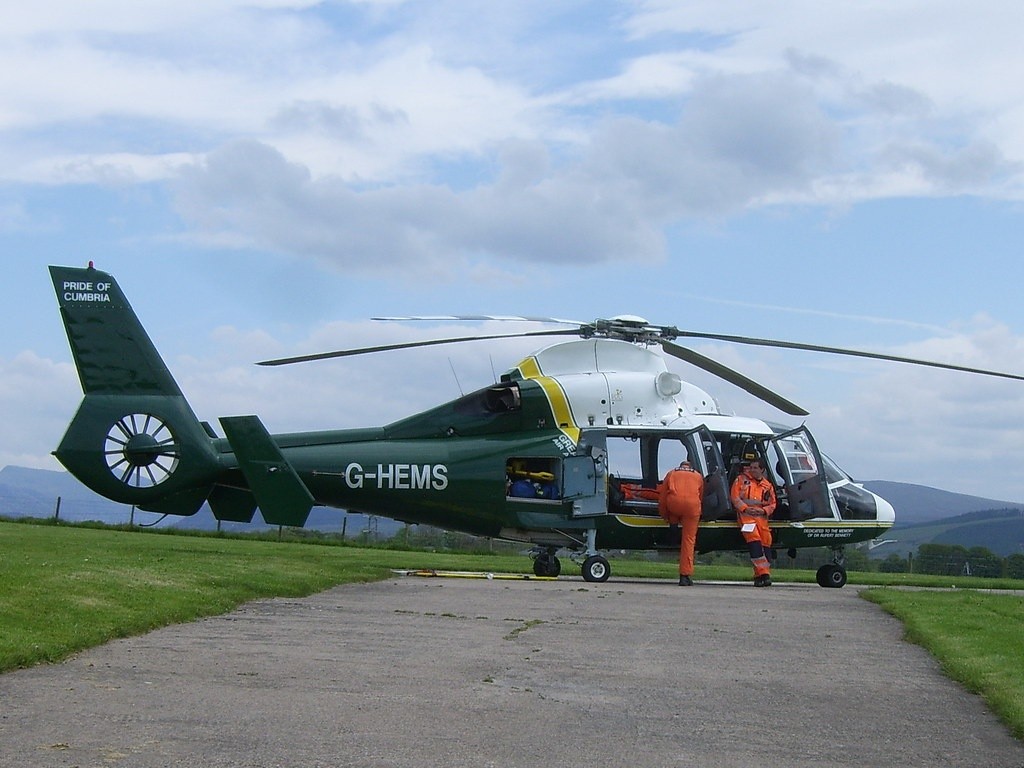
619,484,661,501
659,460,705,586
731,458,777,587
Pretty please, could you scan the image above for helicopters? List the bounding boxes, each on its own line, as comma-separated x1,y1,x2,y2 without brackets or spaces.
47,261,1022,587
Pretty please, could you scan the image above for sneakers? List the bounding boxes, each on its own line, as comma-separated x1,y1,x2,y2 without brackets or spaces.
670,524,678,535
754,577,764,587
761,574,771,586
679,575,693,586
